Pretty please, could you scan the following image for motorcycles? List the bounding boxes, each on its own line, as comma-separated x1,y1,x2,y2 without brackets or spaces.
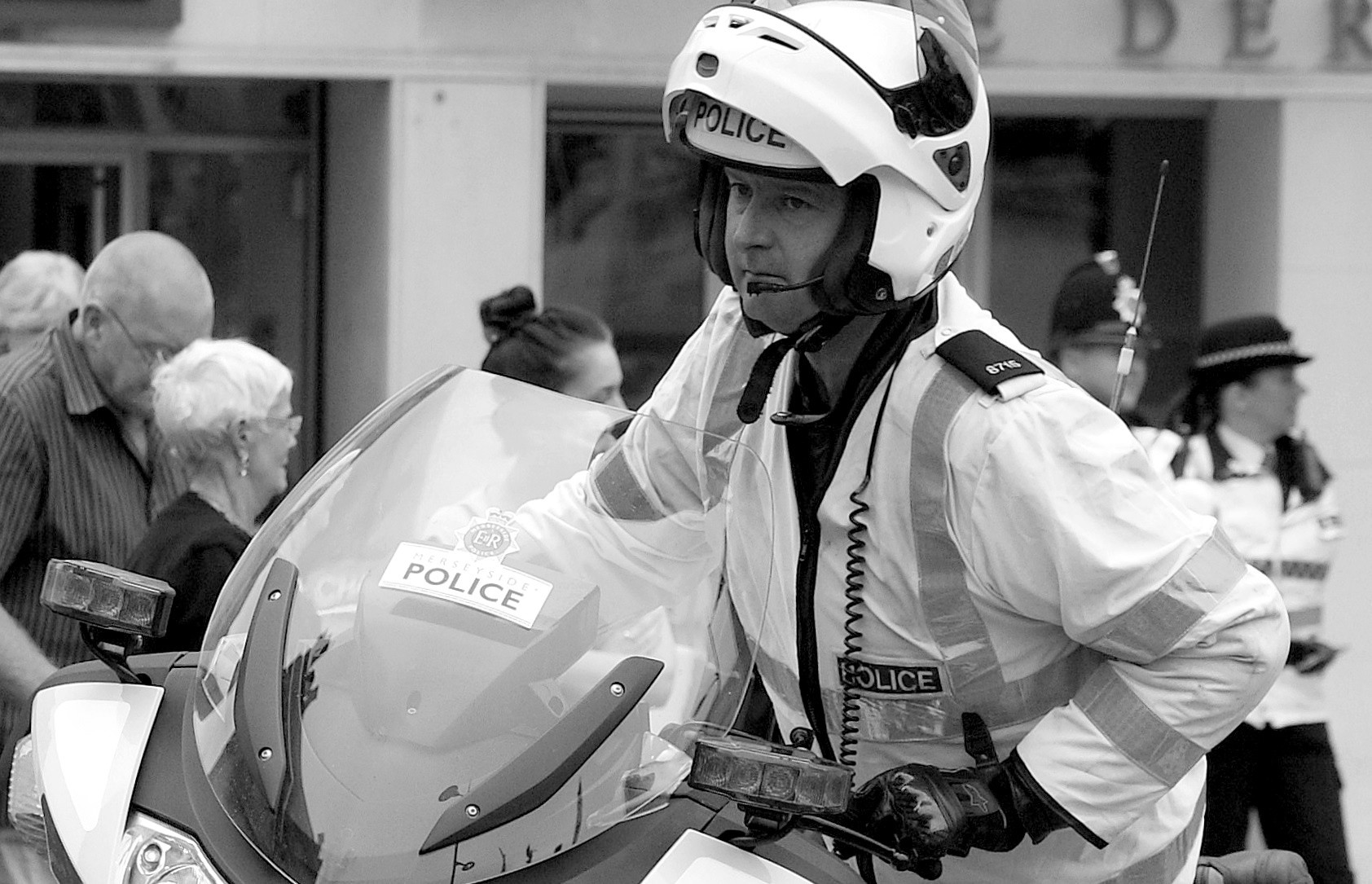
1,359,909,883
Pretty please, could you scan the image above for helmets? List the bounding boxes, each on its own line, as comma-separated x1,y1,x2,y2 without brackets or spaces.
661,0,991,352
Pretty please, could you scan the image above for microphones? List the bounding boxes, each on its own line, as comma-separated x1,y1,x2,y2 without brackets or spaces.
746,274,824,296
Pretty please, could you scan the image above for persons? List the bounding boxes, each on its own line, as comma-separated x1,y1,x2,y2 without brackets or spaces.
424,1,1291,884
0,230,302,884
1038,248,1356,884
424,285,676,708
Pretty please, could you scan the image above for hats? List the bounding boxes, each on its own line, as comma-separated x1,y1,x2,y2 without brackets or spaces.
1187,315,1312,394
1050,250,1160,349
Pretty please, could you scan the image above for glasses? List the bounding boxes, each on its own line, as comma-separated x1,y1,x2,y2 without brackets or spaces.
250,412,304,436
104,306,172,366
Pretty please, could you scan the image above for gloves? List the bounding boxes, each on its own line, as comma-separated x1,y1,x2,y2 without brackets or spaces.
830,712,1071,880
